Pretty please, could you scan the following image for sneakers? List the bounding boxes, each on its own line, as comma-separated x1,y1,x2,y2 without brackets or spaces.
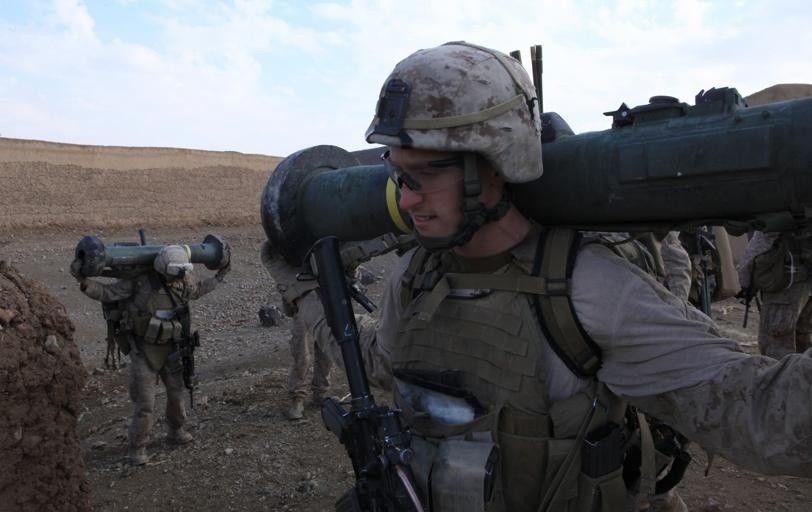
289,400,304,420
312,393,340,406
131,446,150,464
168,427,192,444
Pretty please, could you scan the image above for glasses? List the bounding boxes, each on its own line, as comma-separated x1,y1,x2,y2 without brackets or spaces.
380,148,465,195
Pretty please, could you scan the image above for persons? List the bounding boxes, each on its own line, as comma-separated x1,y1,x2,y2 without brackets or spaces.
261,40,812,512
653,227,693,304
278,295,340,420
739,227,812,358
70,244,231,464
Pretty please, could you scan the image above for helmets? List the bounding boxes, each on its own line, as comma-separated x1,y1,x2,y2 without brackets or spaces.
155,244,193,277
363,39,543,183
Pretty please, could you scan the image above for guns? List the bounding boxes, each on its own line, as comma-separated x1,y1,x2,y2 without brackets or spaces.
733,283,759,327
173,303,201,409
698,235,717,316
295,235,428,512
